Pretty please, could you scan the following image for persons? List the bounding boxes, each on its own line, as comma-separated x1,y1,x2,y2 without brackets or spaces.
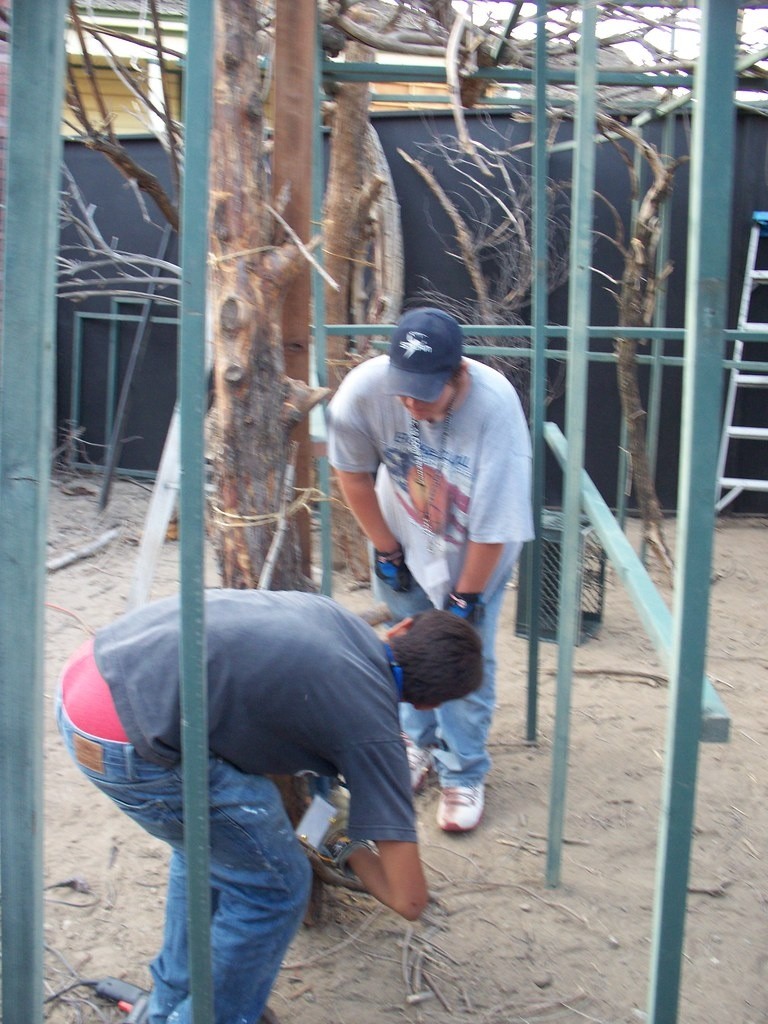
59,586,485,1024
326,308,536,830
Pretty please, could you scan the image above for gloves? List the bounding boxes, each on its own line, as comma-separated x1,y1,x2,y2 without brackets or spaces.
444,587,485,625
374,541,411,593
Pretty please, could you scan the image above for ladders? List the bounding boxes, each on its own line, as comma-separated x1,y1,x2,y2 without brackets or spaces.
712,211,768,515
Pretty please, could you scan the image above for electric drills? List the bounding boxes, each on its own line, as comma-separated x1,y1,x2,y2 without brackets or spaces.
93,974,152,1024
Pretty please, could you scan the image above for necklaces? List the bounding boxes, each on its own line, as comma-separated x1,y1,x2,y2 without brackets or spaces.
411,368,459,555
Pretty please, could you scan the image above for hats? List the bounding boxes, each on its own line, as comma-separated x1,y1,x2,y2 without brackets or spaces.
382,307,462,403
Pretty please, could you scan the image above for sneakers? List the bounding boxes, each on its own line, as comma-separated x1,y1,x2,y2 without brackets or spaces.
436,784,485,831
400,732,439,793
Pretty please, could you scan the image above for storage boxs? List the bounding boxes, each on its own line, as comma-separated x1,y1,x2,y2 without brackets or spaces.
513,509,607,647
